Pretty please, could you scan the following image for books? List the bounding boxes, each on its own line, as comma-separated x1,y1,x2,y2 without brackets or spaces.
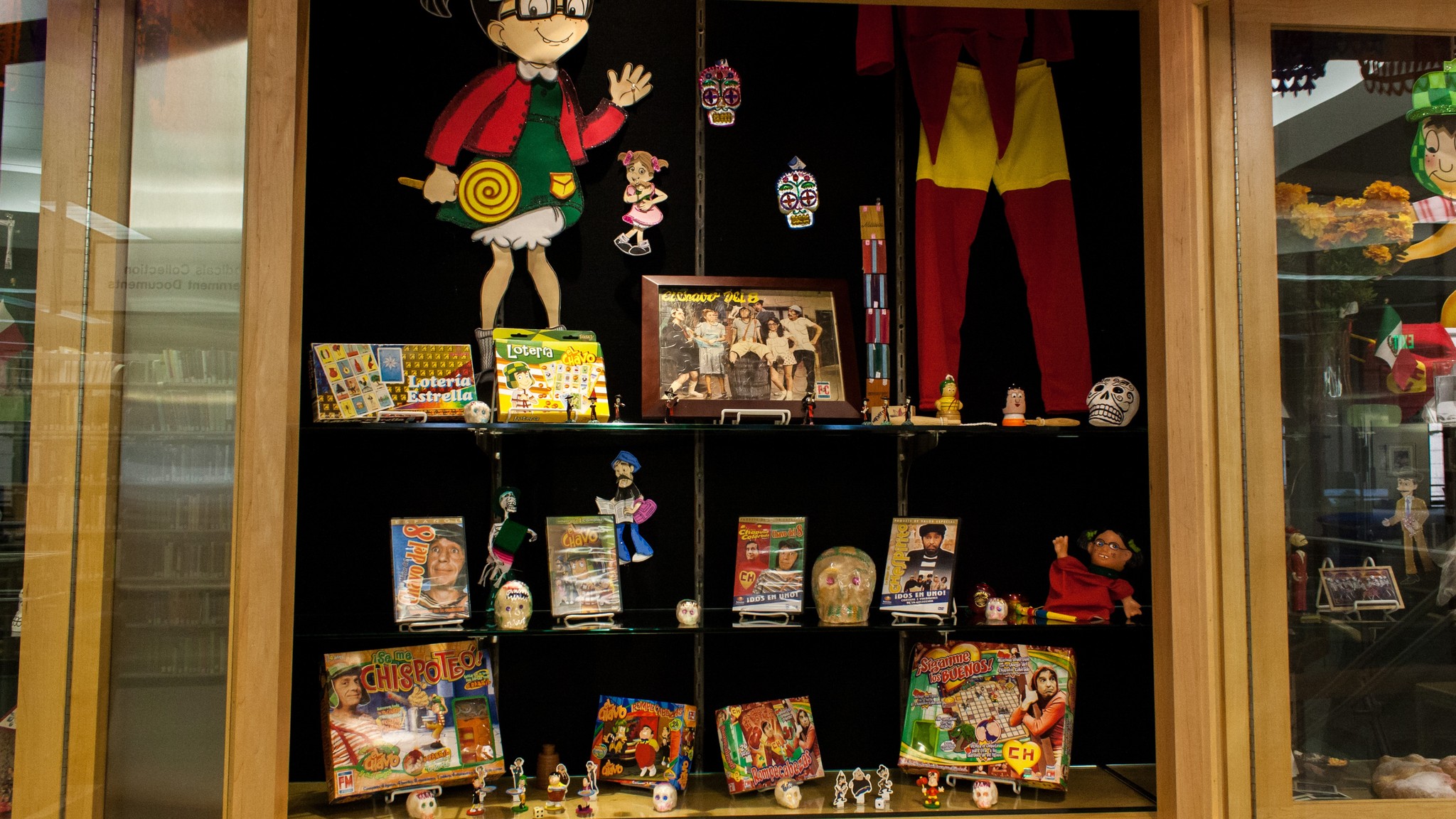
0,346,240,674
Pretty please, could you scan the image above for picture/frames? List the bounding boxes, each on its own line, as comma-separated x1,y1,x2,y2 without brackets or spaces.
1316,563,1408,612
639,272,865,426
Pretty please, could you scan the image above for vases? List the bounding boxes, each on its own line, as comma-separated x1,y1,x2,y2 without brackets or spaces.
1293,314,1357,424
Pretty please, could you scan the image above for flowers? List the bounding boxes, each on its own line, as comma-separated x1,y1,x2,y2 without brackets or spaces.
1273,180,1416,408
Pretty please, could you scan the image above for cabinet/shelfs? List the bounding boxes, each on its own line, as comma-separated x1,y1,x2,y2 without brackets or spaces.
274,0,1456,819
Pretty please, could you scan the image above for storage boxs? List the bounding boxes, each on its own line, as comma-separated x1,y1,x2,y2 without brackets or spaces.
316,636,507,807
714,693,827,798
588,694,699,796
896,640,1078,795
492,326,612,423
306,341,479,424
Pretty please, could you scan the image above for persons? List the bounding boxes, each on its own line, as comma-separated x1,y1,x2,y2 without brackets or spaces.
1052,526,1143,619
661,297,824,403
325,662,390,772
901,525,954,594
1287,533,1310,612
761,709,819,766
397,525,467,616
1008,667,1065,756
1327,574,1393,605
